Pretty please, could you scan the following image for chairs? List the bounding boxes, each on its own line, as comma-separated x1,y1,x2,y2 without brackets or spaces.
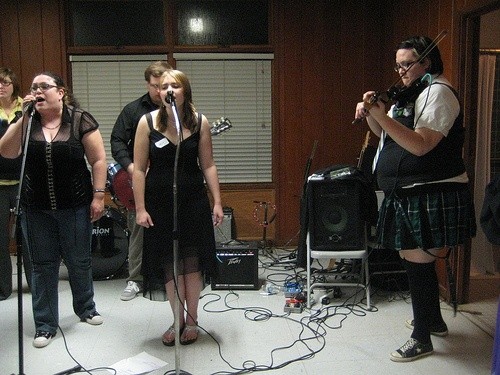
306,233,369,310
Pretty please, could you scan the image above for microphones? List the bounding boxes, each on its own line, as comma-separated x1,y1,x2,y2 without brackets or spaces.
23,98,38,113
165,90,175,104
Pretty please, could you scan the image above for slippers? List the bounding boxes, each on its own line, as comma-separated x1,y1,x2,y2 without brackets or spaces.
162,321,184,347
180,322,200,346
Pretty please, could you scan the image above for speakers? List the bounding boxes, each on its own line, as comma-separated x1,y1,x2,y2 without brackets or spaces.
308,179,364,252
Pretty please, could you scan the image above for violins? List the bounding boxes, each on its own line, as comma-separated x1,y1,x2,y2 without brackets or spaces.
370,80,426,106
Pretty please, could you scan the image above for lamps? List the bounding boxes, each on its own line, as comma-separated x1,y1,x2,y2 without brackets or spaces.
189,17,203,32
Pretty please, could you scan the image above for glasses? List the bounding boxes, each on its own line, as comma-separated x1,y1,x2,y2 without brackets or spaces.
0,80,14,87
28,83,61,93
392,60,419,72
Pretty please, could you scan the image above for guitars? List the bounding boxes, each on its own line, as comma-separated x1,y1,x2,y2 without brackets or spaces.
104,117,232,210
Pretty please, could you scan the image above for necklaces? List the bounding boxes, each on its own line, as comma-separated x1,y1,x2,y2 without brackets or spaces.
39,120,63,130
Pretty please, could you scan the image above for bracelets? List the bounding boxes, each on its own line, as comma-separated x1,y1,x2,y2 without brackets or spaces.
92,188,107,194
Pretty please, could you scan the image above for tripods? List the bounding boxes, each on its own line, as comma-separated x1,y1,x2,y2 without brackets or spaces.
9,109,81,375
258,205,281,264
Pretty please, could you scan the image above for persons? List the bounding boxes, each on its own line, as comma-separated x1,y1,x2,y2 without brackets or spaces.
109,60,175,301
0,71,107,350
0,67,37,302
354,34,480,363
132,69,226,348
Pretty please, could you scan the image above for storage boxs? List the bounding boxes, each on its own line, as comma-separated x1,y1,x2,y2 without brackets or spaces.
211,244,258,290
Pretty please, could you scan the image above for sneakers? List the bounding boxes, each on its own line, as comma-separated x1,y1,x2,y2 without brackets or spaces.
405,318,450,337
83,310,104,325
121,280,144,299
33,329,54,348
390,337,434,362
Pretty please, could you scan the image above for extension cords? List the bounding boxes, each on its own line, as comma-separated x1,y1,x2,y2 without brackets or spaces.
284,290,300,297
284,303,303,313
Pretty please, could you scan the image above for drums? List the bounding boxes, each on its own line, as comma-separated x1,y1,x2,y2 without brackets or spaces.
76,206,128,279
